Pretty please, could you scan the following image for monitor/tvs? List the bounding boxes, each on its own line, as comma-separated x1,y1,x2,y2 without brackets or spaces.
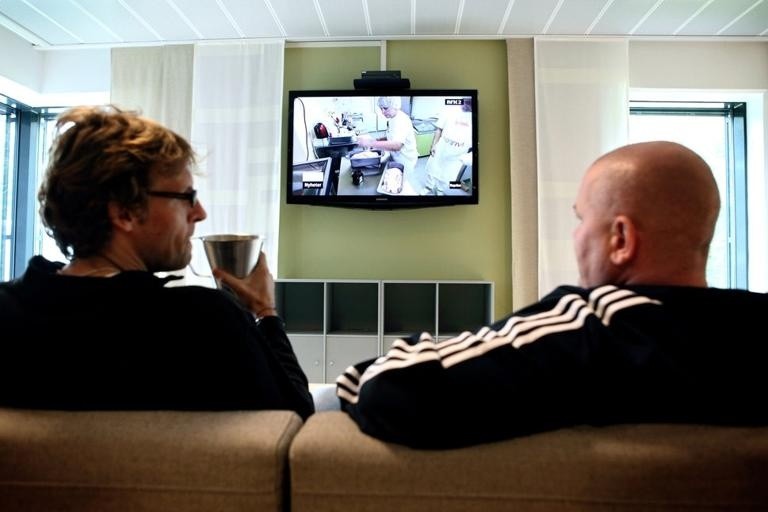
286,89,479,212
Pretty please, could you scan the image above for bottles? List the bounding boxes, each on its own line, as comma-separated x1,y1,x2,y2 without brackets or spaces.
352,169,364,185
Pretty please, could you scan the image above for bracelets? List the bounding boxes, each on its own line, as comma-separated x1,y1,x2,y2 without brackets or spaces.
257,306,277,316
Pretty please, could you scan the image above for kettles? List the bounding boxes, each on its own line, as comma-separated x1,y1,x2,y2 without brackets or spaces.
314,122,328,139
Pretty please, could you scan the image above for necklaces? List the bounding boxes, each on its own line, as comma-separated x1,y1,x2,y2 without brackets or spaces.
55,266,115,277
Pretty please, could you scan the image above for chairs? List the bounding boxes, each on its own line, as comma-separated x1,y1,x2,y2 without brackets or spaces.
3,407,306,510
290,411,768,510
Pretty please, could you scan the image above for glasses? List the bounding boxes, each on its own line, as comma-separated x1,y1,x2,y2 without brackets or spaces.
147,190,198,208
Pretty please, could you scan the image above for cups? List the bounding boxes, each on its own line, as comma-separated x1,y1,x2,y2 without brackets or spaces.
188,235,264,325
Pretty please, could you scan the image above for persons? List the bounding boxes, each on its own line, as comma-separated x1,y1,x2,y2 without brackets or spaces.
358,96,419,195
420,96,472,195
337,142,767,452
0,106,314,420
